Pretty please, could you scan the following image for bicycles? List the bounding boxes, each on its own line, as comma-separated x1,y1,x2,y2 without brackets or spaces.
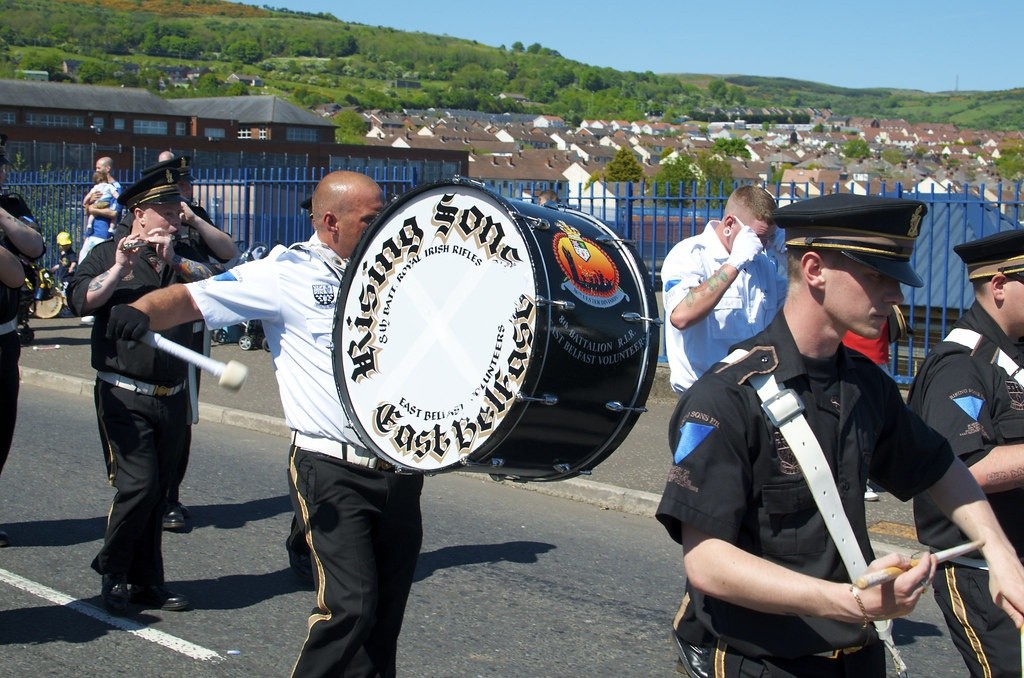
30,288,68,318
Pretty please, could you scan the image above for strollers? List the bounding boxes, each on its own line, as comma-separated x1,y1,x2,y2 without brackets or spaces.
212,243,270,352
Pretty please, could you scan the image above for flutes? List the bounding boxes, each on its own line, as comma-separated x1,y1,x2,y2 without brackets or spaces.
122,233,191,251
190,201,197,205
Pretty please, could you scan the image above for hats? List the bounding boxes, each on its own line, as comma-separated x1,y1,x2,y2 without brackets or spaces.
953,229,1024,284
773,193,928,288
117,167,190,210
56,232,71,246
141,156,196,182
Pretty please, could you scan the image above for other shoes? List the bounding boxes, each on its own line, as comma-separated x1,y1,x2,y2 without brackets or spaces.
286,542,313,582
81,316,95,322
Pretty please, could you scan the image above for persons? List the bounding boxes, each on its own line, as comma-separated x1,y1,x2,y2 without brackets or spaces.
660,185,787,397
0,152,237,616
905,228,1024,678
108,169,425,678
841,302,889,502
537,190,561,205
285,195,314,585
655,194,1024,678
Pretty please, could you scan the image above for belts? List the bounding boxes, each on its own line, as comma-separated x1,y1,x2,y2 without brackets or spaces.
290,429,395,470
928,546,990,571
97,370,187,397
0,315,18,335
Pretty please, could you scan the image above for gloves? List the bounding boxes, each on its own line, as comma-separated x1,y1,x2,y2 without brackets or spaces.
104,304,149,350
726,224,766,272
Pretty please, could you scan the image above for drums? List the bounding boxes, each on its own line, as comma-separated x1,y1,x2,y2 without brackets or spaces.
330,173,664,482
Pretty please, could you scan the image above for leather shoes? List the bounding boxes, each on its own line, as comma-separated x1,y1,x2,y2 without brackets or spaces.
162,498,189,528
672,630,710,678
101,572,190,617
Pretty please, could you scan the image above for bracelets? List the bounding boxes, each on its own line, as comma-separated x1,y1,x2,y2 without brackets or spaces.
849,584,870,629
